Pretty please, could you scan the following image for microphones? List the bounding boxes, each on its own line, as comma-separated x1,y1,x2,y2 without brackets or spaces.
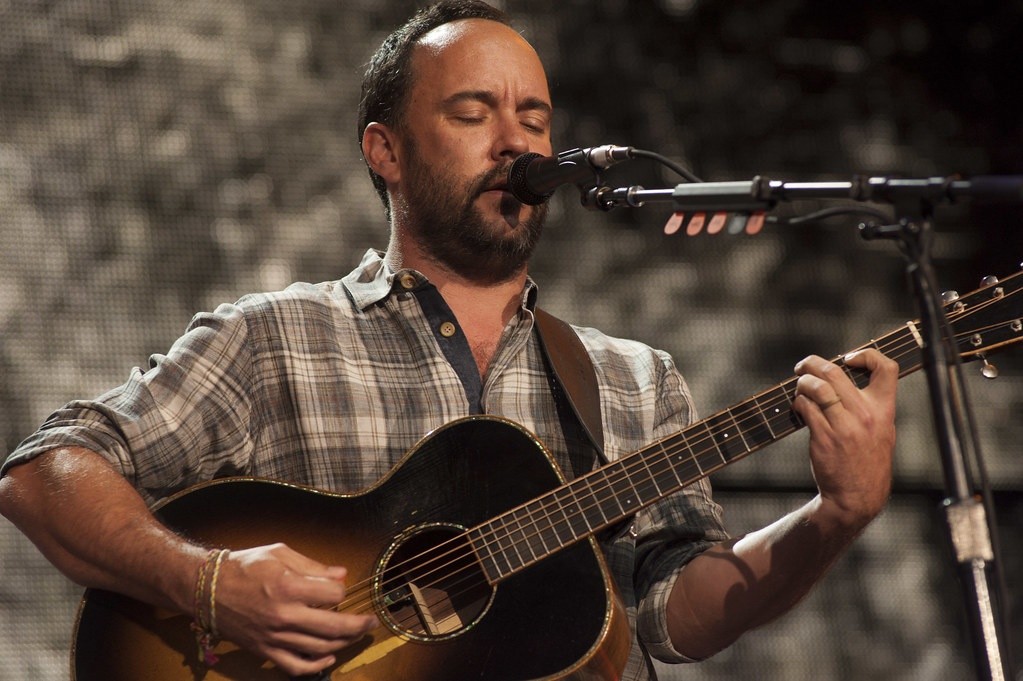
506,145,631,206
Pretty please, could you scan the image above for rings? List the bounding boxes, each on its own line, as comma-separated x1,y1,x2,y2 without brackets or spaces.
820,395,841,410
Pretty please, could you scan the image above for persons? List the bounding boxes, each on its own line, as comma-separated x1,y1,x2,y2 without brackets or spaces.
0,0,898,681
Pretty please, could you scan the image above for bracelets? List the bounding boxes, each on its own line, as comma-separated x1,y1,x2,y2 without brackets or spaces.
191,548,230,665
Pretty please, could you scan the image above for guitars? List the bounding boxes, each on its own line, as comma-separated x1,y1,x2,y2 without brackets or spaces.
65,262,1023,681
